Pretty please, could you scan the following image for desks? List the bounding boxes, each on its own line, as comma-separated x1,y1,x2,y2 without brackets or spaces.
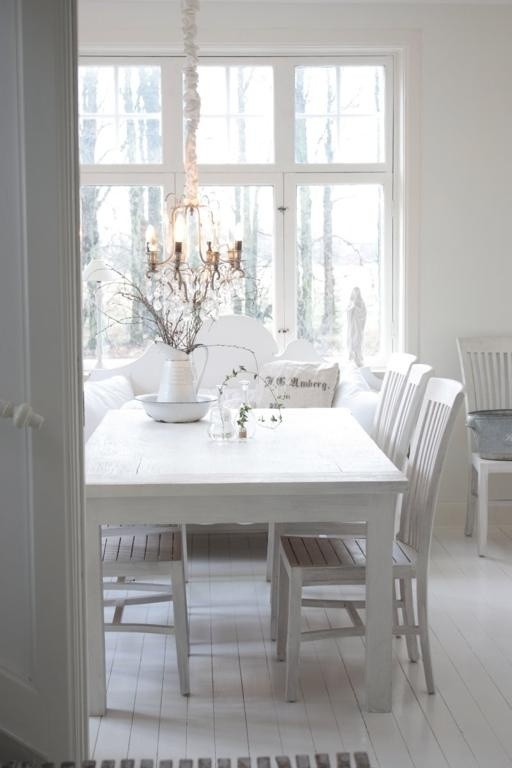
82,408,411,711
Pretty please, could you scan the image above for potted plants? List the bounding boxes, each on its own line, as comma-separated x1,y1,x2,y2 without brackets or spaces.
81,255,254,403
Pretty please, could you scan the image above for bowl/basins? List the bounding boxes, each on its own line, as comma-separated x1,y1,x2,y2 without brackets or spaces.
135,394,218,423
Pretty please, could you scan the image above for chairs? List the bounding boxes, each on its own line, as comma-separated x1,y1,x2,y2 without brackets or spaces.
454,336,512,560
261,349,418,582
94,526,193,698
250,361,436,641
270,375,468,704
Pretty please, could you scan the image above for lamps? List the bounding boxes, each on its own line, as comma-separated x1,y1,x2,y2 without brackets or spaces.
143,0,250,283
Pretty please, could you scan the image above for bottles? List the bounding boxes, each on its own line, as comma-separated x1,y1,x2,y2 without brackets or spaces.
207,380,257,443
